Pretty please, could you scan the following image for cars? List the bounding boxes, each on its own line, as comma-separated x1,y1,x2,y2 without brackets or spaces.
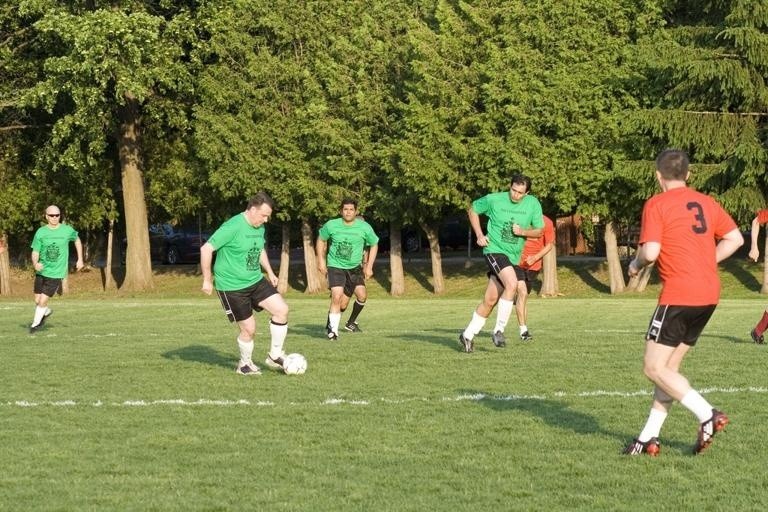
118,222,210,265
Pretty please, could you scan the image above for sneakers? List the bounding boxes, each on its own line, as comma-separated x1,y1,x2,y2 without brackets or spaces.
265,352,285,370
622,437,661,457
328,332,338,342
491,330,505,348
751,328,766,345
344,321,362,333
693,408,729,455
520,331,532,342
235,360,262,376
460,333,473,354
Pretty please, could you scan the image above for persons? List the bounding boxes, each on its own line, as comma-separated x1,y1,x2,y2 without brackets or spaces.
200,193,289,376
748,209,768,345
30,205,85,333
619,149,745,457
459,174,545,354
316,197,380,343
515,213,555,342
326,216,367,329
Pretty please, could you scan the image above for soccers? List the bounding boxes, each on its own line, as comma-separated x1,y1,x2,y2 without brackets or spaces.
283,353,307,375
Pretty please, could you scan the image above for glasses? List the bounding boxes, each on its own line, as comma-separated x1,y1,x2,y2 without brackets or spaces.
47,213,60,217
